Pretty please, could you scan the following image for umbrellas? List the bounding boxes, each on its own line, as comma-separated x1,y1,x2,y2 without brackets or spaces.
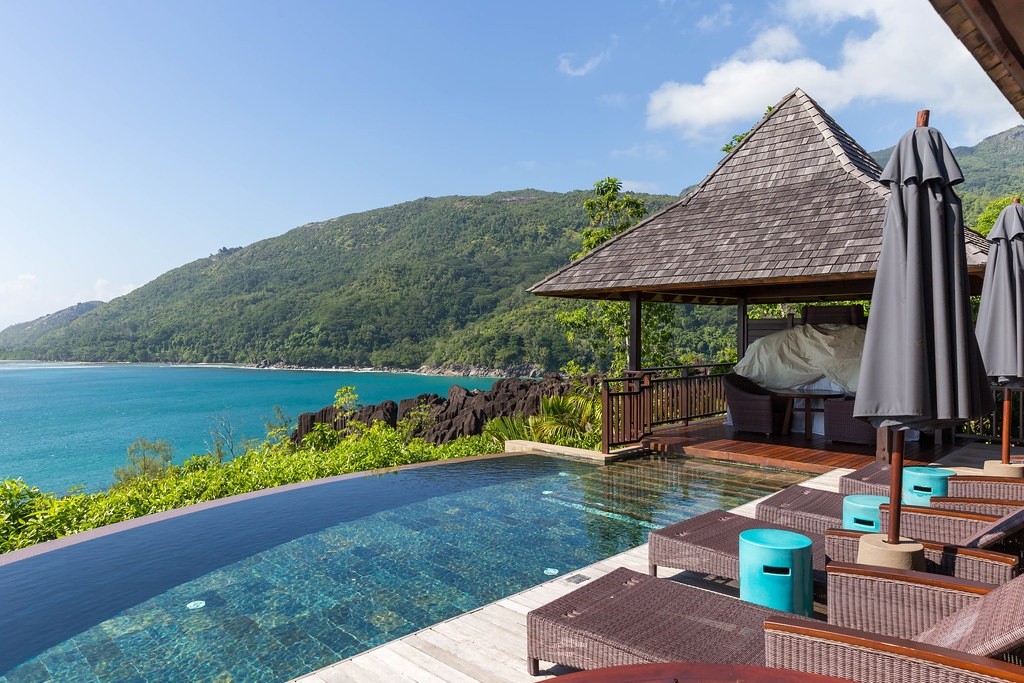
852,110,1024,543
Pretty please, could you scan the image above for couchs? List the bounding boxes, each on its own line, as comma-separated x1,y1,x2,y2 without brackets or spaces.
824,396,877,447
722,373,793,437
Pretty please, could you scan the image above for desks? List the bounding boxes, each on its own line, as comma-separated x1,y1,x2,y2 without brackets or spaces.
773,389,845,440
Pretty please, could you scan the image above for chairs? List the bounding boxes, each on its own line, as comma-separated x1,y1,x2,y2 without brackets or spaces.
651,505,1024,606
840,460,1024,501
527,560,1024,683
757,484,1024,533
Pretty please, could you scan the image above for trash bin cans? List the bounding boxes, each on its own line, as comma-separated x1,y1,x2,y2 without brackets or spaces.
738,527,814,622
901,466,958,507
843,494,907,533
